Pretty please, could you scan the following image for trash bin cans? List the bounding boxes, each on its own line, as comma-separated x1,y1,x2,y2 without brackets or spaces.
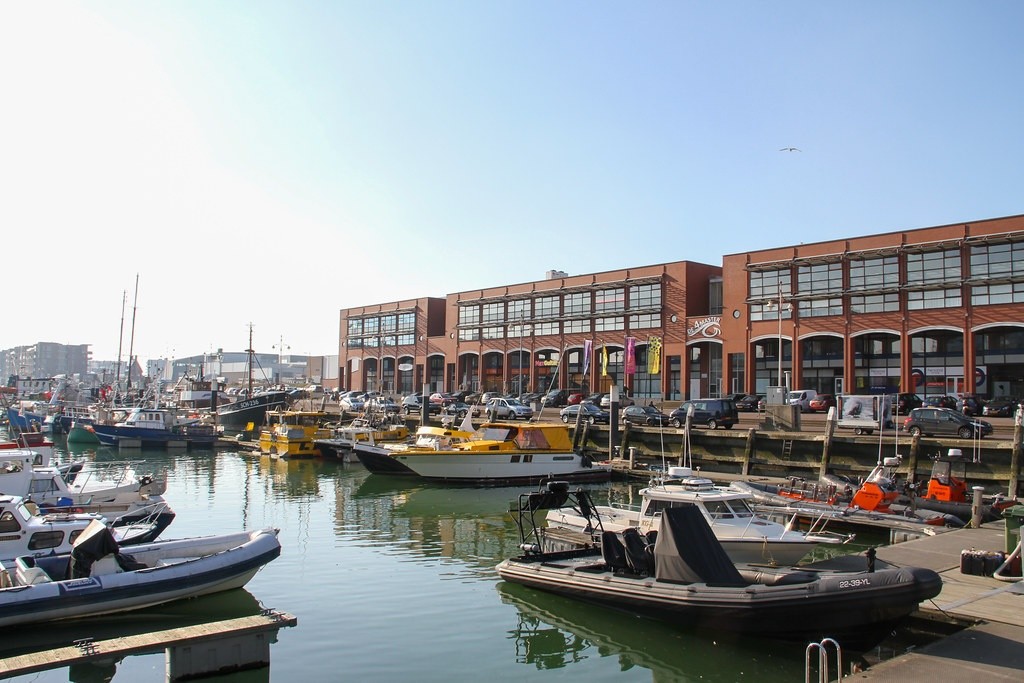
1000,505,1024,555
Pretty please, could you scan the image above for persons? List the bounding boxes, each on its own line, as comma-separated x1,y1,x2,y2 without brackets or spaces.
388,396,393,402
958,394,972,416
623,384,629,395
649,401,655,408
364,393,369,398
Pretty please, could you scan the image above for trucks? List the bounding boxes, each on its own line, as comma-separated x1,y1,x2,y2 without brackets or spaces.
838,394,893,435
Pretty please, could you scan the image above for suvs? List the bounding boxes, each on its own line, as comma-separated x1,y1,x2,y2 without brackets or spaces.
621,405,670,427
484,397,533,420
903,407,994,439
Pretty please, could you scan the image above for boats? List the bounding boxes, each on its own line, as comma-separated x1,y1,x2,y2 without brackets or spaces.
255,344,613,480
495,581,851,683
495,406,944,631
0,273,293,627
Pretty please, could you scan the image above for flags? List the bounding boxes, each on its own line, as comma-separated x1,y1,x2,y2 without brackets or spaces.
583,340,592,376
602,341,608,376
648,336,660,375
18,403,25,418
625,337,636,374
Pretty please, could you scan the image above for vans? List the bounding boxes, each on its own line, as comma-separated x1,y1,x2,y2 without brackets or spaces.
785,390,817,411
669,398,739,429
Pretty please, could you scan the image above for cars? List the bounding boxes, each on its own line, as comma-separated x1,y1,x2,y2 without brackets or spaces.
225,383,483,417
890,392,1024,418
723,392,767,412
810,394,837,414
481,392,636,408
560,405,610,425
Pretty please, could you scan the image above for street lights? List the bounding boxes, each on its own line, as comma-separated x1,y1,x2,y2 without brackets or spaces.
272,335,291,384
766,281,795,388
372,323,395,397
505,309,535,402
